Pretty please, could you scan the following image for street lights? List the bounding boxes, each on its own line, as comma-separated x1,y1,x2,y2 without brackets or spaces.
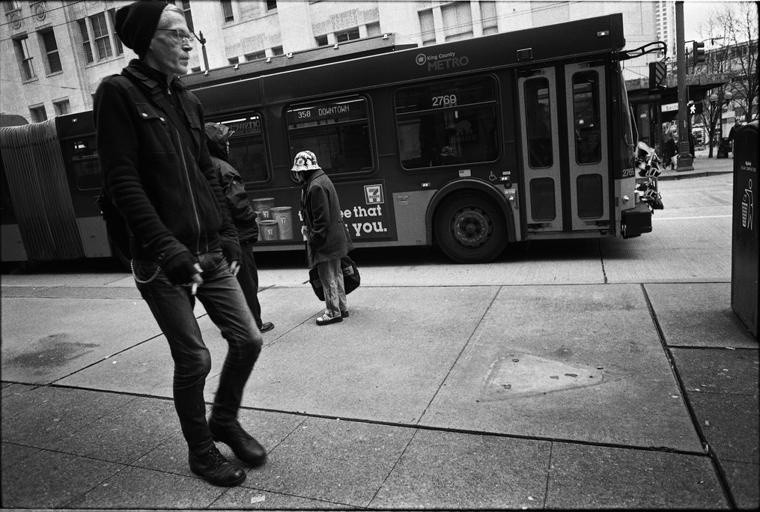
710,92,732,156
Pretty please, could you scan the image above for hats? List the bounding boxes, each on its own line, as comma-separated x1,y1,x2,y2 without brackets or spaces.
290,150,322,172
204,121,236,144
114,0,170,60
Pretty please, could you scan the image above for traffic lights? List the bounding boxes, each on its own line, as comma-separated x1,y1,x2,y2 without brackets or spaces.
693,41,705,64
688,102,703,116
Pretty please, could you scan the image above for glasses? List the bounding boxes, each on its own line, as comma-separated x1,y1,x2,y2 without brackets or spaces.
153,29,195,43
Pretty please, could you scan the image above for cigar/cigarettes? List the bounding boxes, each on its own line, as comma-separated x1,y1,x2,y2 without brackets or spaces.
191,280,197,296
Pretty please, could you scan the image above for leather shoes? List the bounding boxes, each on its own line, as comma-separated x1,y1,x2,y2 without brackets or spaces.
189,421,268,488
260,322,274,333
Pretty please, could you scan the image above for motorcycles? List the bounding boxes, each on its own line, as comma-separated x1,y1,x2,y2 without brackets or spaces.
717,138,728,160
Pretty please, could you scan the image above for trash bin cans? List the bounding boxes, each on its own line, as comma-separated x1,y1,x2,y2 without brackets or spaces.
731,120,760,339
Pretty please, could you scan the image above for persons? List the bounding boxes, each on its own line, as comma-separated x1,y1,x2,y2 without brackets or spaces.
291,151,353,326
728,118,742,158
203,122,274,338
91,1,268,486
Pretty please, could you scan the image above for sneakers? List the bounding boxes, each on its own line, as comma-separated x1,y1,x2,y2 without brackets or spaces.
315,309,349,326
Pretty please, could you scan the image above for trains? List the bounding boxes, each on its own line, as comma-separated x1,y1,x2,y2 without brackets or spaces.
0,13,671,272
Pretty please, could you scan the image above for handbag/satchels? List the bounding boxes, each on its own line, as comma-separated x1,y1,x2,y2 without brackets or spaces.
309,255,361,302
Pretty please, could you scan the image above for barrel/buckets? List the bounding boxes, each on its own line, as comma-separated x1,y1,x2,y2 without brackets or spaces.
268,207,293,241
254,208,268,226
252,198,274,212
260,219,279,241
